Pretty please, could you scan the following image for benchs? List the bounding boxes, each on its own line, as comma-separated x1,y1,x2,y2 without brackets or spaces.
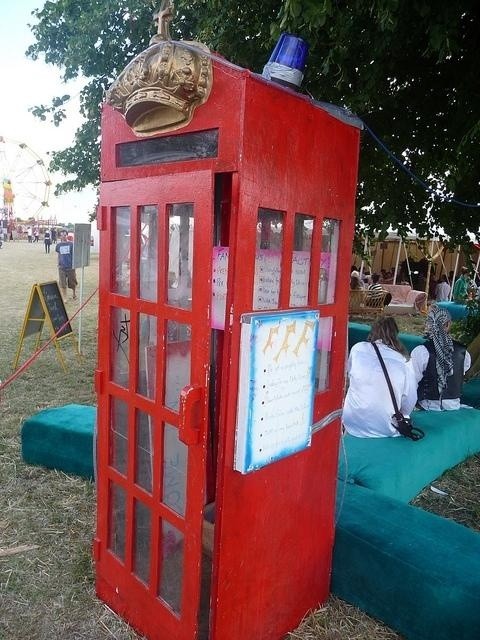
335,403,480,505
22,403,96,479
331,480,480,640
349,290,389,321
348,322,430,355
435,301,478,322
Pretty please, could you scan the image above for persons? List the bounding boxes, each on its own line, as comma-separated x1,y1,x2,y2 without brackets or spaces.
54,230,78,300
350,252,480,306
0,216,78,254
342,316,419,440
409,308,475,412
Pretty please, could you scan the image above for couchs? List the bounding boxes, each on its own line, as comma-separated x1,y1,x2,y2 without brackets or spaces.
364,283,428,315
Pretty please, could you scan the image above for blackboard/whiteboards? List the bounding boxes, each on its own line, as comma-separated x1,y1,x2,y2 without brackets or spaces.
20,284,45,338
36,280,74,341
